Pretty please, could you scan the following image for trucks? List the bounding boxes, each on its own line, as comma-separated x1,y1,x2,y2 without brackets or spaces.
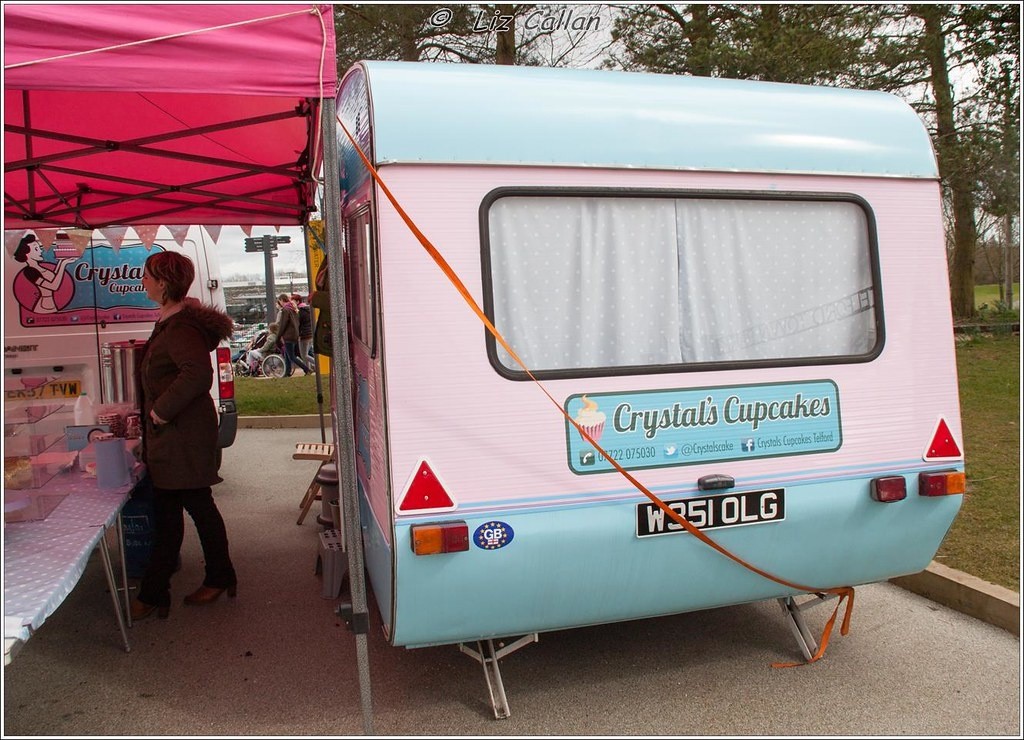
5,223,238,449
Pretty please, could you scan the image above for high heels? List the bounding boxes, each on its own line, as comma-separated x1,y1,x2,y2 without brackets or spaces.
185,577,236,602
124,592,171,620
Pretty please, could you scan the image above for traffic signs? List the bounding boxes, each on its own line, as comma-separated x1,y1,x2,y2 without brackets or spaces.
244,236,264,253
274,236,291,244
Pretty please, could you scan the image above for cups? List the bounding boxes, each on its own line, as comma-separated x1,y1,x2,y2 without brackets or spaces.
127,416,138,437
94,433,114,440
93,438,131,489
98,413,119,437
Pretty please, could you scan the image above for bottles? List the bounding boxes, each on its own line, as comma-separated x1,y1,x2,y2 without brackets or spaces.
74,392,96,425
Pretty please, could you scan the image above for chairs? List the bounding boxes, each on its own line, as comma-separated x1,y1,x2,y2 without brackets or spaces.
292,443,334,526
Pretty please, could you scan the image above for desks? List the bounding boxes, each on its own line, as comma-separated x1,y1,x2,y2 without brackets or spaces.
4,435,145,666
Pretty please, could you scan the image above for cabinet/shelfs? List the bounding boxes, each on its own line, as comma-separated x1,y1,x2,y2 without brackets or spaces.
4,407,75,523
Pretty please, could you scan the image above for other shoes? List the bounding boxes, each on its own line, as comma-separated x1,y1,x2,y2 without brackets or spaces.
304,369,313,376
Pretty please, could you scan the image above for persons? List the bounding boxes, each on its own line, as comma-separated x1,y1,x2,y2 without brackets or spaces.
231,253,332,376
116,251,237,619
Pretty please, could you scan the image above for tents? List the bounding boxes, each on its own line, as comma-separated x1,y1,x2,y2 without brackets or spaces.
5,4,372,735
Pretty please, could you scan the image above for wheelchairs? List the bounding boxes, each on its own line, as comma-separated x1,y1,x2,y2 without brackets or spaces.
241,342,296,379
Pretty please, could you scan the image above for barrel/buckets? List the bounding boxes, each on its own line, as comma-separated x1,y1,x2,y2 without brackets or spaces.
327,498,341,529
